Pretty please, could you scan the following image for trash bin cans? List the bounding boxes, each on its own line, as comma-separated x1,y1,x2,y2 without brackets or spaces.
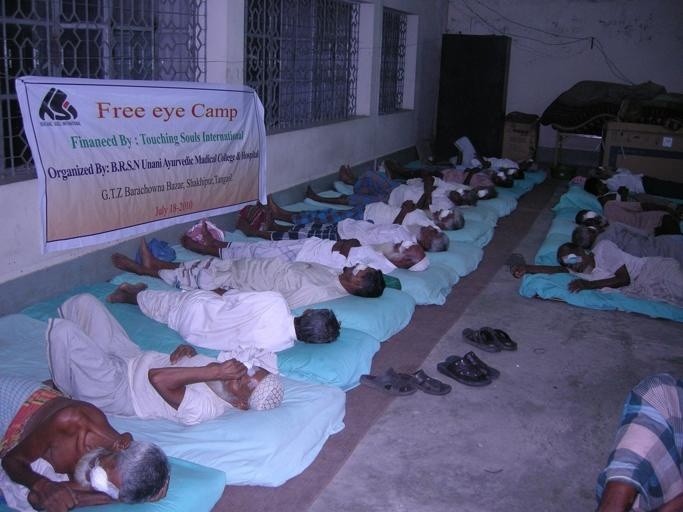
501,111,540,161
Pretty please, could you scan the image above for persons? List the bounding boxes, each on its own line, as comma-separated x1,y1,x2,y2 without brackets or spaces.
591,369,681,510
0,373,170,510
106,283,340,353
109,235,383,312
41,292,285,427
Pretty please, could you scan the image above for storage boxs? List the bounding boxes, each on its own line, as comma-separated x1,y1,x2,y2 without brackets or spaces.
501,118,539,162
601,121,683,184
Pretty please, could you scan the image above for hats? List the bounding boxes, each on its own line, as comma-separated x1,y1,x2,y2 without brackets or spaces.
410,255,429,272
248,373,284,411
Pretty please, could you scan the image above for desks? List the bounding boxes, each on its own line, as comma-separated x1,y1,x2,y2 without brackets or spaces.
552,131,602,168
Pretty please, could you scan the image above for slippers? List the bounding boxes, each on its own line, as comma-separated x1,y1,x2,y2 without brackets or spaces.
360,351,500,394
462,326,518,352
